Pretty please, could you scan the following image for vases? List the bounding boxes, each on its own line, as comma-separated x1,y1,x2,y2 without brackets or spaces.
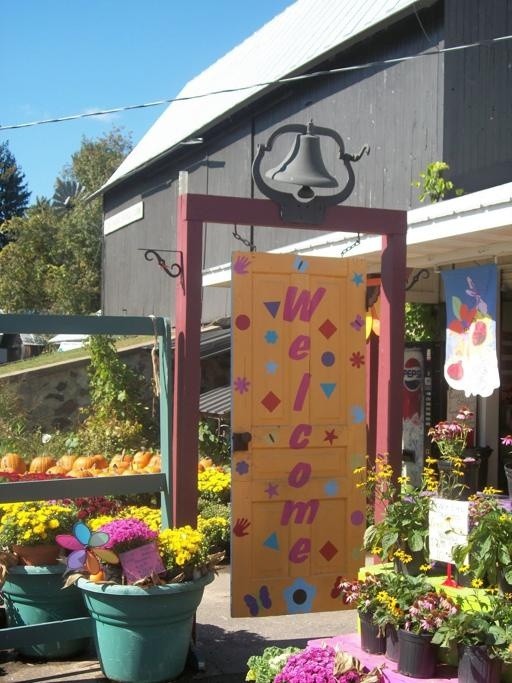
387,531,507,589
1,557,216,682
352,601,504,682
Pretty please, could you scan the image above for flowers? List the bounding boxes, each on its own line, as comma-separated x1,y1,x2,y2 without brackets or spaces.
0,458,236,584
334,399,512,652
243,642,387,682
